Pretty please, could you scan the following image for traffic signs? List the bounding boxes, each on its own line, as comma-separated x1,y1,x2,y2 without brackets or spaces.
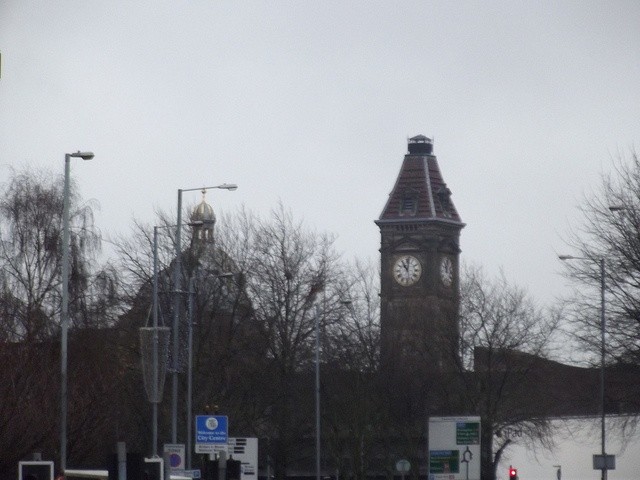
194,414,229,456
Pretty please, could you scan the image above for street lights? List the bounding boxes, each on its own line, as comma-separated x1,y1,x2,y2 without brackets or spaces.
179,184,236,479
552,253,606,479
61,148,96,477
313,294,352,479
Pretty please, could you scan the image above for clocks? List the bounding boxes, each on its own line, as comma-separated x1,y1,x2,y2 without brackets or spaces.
439,256,454,288
392,255,423,287
